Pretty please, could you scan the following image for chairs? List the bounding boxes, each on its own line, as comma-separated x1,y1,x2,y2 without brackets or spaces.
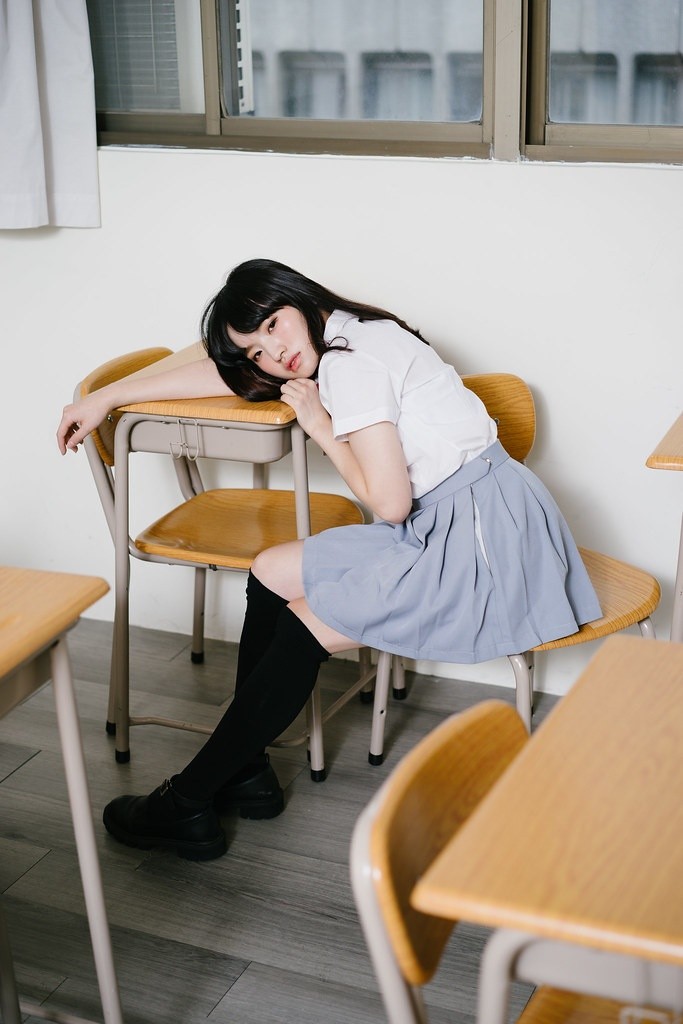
349,699,683,1024
367,373,663,767
73,346,374,762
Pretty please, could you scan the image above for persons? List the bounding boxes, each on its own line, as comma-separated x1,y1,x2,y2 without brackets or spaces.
56,260,602,860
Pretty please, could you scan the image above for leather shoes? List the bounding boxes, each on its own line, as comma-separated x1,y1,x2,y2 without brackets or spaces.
212,752,285,821
103,777,229,861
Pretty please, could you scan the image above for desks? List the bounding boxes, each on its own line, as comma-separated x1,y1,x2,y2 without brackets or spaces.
403,632,682,1024
645,411,683,643
113,397,376,784
0,567,127,1024
